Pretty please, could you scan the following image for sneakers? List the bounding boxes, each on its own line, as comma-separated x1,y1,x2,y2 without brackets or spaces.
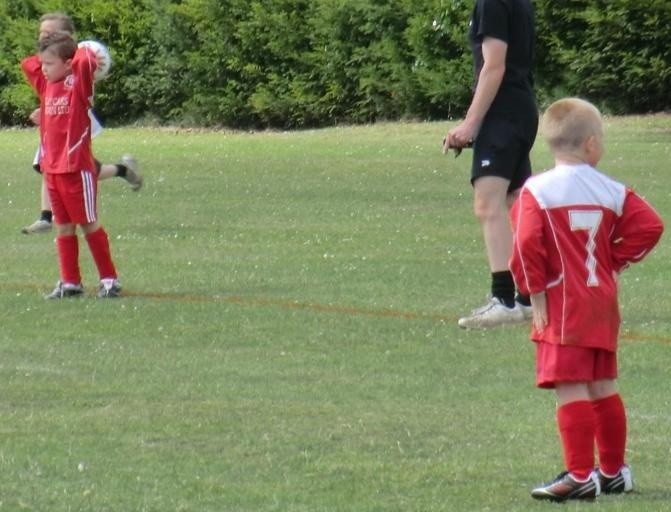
21,219,52,234
531,465,632,500
98,278,121,298
122,154,142,190
457,296,532,329
44,281,83,300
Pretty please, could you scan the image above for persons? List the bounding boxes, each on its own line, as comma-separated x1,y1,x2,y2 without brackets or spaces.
20,13,142,301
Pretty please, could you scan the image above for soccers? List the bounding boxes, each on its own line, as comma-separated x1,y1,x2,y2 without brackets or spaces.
76,41,111,82
